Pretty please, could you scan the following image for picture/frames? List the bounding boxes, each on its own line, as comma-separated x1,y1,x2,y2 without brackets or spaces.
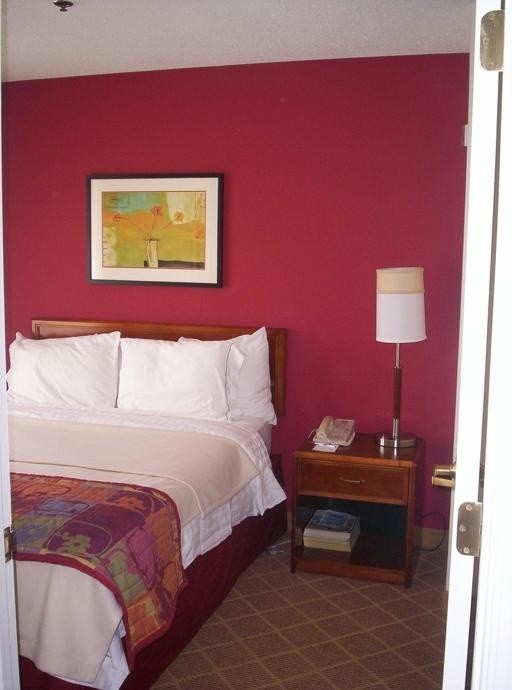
86,174,222,287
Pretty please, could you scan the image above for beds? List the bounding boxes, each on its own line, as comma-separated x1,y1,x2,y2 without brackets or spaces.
5,317,286,690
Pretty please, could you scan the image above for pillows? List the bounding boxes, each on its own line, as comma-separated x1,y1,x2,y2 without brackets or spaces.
11,332,120,406
115,340,230,418
178,327,277,425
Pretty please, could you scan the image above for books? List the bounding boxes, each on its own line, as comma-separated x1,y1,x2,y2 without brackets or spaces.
303,507,362,551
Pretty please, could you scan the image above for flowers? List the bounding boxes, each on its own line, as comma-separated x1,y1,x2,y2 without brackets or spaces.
112,205,184,242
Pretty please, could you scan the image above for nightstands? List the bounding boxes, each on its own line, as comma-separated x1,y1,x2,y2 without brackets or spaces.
289,431,425,591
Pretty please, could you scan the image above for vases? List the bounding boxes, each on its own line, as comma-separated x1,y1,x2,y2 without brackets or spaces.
143,239,160,269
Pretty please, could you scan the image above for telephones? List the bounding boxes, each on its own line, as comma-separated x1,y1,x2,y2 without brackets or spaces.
313,416,356,446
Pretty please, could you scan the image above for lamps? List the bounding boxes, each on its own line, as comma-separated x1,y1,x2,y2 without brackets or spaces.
375,265,428,449
